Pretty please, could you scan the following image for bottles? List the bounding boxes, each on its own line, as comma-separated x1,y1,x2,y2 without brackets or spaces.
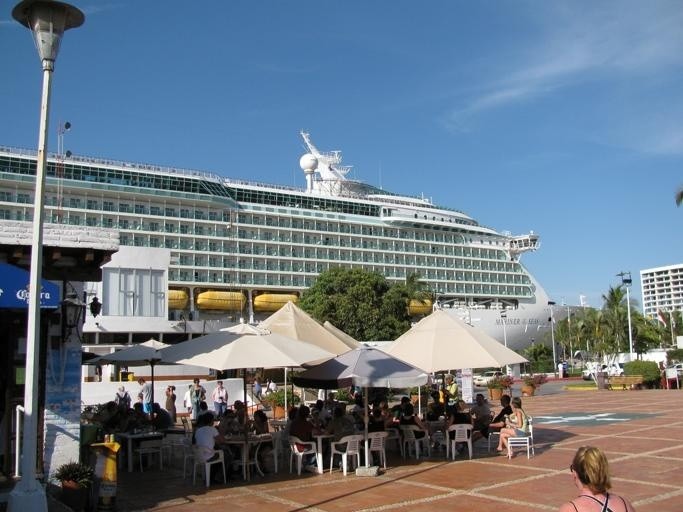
506,417,511,430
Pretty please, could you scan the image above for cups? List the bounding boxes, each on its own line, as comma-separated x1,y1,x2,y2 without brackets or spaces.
110,434,115,443
103,435,109,443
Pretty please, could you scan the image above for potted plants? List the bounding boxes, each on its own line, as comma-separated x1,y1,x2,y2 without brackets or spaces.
49,461,96,504
264,388,296,420
410,382,428,407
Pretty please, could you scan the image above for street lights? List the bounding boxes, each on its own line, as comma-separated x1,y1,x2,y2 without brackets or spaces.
616,271,634,361
8,2,86,511
549,301,558,371
500,314,509,346
55,118,74,223
531,336,537,346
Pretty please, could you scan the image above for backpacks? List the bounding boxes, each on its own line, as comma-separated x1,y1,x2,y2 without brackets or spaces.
117,392,127,409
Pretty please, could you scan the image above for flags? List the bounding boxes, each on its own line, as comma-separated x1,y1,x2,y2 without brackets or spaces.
657,308,667,328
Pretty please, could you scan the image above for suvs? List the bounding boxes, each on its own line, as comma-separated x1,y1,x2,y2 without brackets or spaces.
665,363,683,377
611,362,626,376
582,363,609,380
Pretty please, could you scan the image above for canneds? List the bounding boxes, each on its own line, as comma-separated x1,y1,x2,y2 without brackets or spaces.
103,434,115,442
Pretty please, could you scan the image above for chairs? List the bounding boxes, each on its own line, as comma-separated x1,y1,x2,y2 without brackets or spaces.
116,406,534,488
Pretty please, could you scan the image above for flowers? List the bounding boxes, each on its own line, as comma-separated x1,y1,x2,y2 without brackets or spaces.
520,373,548,387
486,374,514,389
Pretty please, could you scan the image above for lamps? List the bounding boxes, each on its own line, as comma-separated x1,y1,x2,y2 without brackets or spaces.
85,292,103,318
58,287,84,344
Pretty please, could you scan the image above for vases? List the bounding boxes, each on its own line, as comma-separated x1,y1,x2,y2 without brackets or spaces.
488,388,502,402
522,384,533,398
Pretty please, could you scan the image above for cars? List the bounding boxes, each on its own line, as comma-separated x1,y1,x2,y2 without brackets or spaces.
431,374,454,386
475,371,506,387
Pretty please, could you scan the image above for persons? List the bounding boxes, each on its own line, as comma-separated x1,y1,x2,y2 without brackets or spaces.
557,362,563,378
558,446,636,512
563,361,568,377
660,365,667,389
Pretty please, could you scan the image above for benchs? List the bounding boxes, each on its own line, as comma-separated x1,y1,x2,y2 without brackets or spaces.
605,375,644,390
